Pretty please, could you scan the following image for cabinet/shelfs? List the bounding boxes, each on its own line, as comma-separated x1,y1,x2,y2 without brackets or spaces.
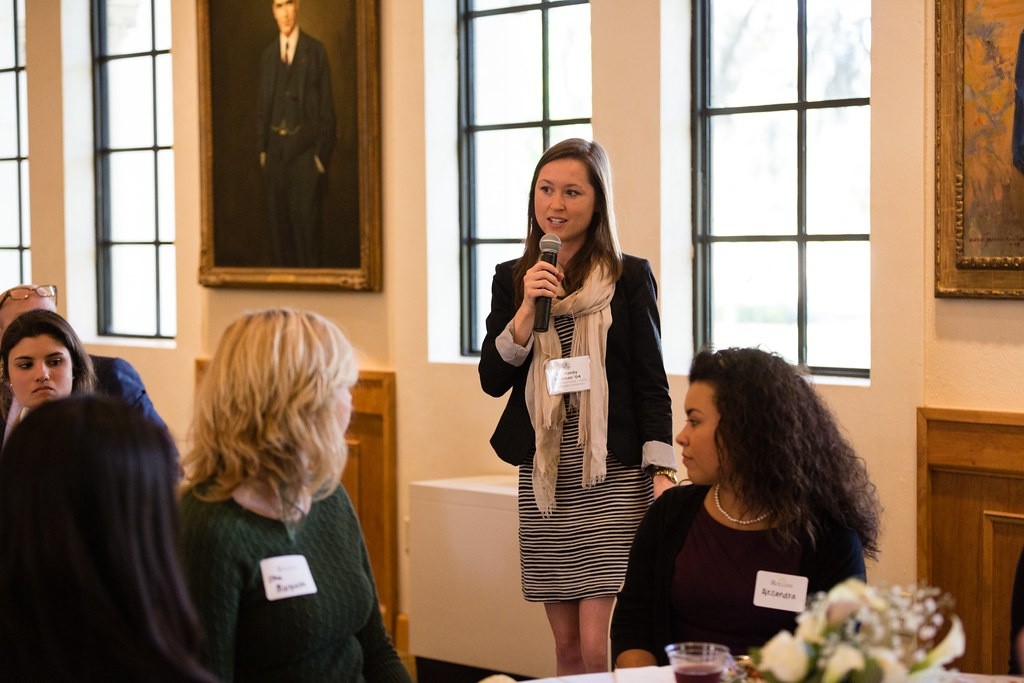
406,477,558,682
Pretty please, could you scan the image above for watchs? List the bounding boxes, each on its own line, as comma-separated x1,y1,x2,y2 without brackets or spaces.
652,469,678,484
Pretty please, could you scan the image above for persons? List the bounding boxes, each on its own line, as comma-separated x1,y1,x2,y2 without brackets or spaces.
254,0,336,267
0,285,218,683
178,308,414,683
478,137,681,677
610,348,884,673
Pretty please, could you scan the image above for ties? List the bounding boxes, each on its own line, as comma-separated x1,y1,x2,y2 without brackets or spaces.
285,42,289,64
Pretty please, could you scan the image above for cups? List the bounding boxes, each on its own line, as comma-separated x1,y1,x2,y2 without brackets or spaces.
664,640,731,683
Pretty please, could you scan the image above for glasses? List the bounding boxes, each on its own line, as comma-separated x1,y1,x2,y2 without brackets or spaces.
0,285,58,309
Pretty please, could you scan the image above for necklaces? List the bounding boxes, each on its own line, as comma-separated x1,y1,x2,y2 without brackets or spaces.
714,483,774,525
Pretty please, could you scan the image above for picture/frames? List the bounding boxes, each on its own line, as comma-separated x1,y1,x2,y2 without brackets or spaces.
935,0,1024,296
194,0,379,290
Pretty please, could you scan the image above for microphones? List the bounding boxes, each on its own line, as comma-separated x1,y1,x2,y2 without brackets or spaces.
533,233,562,333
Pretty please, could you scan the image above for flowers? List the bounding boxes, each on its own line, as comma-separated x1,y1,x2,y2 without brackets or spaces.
742,576,968,683
